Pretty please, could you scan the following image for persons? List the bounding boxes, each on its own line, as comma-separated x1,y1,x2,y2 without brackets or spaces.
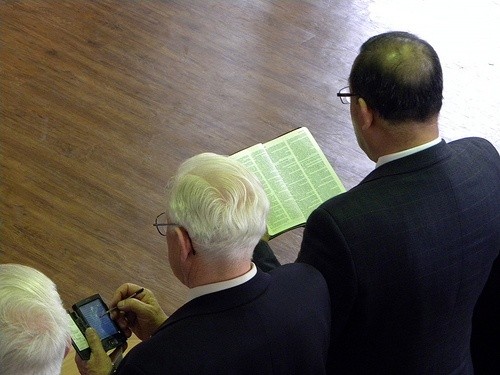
75,152,335,375
294,32,500,375
0,264,72,375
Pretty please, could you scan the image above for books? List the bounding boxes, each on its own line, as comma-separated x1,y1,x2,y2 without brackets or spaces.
230,126,348,242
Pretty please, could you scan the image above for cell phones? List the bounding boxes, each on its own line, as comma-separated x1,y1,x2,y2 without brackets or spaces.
72,293,126,352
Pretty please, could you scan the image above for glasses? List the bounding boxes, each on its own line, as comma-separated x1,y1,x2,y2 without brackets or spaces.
337,86,375,114
152,212,195,256
